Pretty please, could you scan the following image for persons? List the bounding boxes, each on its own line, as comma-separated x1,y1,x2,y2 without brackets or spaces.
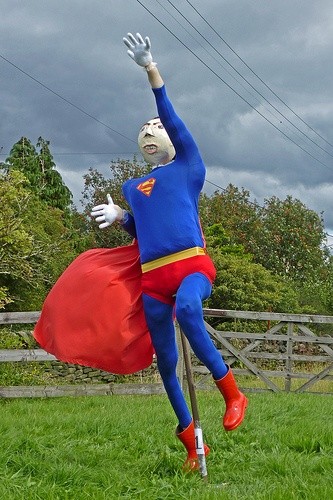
91,31,249,470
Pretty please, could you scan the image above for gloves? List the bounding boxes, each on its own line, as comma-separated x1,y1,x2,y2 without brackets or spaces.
90,193,122,229
123,32,157,72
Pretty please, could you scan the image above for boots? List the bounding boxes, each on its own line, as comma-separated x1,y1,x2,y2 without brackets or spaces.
211,363,248,431
175,418,211,474
